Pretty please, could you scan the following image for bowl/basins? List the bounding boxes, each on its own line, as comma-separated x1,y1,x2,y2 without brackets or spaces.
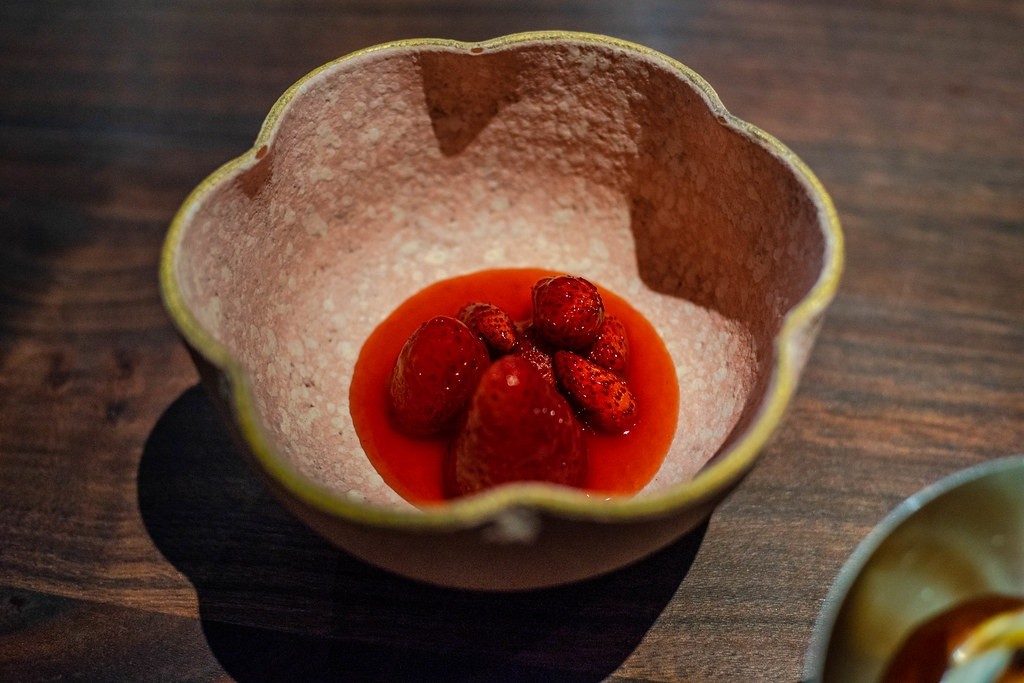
159,33,844,574
800,457,1024,683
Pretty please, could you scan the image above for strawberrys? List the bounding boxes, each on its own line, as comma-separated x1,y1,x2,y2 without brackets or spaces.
389,273,636,497
